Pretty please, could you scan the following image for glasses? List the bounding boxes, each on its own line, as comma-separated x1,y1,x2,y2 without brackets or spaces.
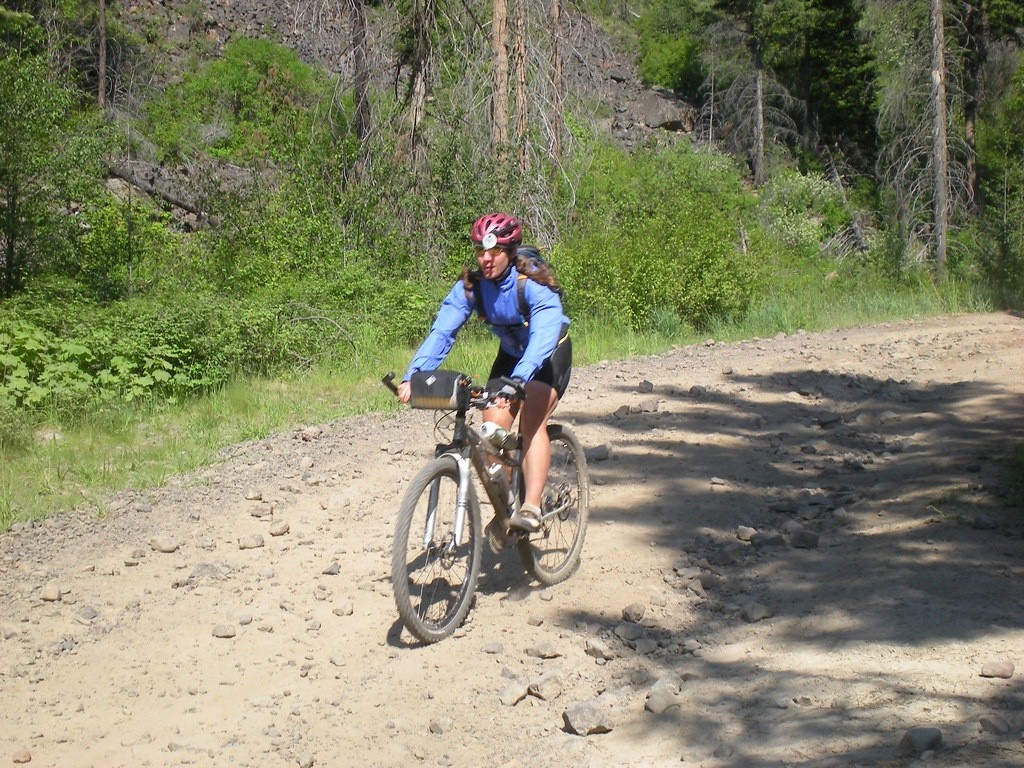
473,244,510,257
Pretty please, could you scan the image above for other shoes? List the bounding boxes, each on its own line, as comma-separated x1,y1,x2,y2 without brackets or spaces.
509,504,542,532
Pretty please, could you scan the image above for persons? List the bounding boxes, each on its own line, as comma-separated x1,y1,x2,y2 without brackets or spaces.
396,213,572,535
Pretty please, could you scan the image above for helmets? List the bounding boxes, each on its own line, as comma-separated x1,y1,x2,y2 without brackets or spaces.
470,213,523,249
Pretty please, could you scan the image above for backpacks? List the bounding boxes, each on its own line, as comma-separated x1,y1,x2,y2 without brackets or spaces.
473,245,562,333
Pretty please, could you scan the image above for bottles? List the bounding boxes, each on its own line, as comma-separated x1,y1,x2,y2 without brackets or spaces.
488,462,515,507
478,422,516,450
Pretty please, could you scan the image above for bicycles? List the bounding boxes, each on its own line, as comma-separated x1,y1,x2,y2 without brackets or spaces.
381,370,591,644
